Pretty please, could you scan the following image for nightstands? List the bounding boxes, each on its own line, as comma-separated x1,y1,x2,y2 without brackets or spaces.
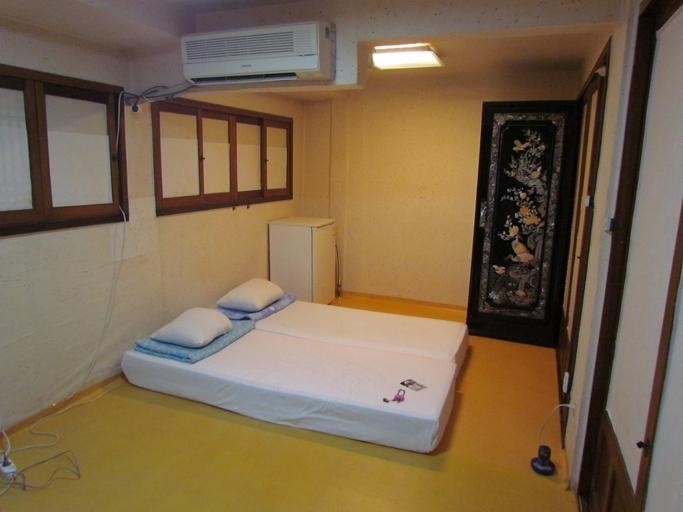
265,215,337,305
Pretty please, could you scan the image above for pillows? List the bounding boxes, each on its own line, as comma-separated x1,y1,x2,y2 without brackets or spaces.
216,276,285,312
148,308,233,348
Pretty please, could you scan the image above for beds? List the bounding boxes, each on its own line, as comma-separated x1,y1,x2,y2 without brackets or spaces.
209,299,471,372
122,329,460,453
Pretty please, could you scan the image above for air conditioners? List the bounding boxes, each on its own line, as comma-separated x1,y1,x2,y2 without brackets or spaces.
182,18,338,87
